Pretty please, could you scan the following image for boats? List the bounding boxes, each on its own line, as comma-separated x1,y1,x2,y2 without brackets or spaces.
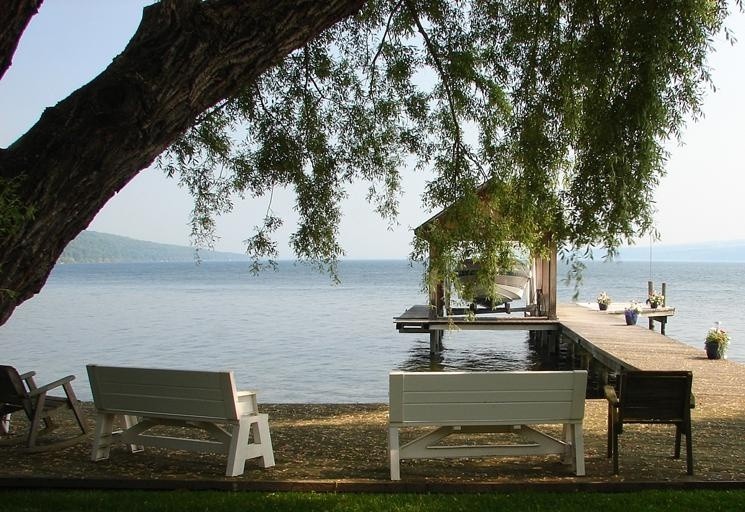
453,258,532,303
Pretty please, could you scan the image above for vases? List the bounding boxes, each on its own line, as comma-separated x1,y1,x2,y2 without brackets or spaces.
599,302,607,310
624,311,638,324
706,341,722,359
651,303,659,308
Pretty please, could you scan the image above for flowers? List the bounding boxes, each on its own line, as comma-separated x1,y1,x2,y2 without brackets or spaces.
597,291,612,306
704,326,731,360
646,289,665,305
623,300,643,315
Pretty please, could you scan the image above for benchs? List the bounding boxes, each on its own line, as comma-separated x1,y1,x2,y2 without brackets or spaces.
89,365,276,478
389,368,589,478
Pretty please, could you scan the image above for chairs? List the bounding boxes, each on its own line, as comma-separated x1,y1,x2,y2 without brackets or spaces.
605,370,696,476
0,364,96,456
